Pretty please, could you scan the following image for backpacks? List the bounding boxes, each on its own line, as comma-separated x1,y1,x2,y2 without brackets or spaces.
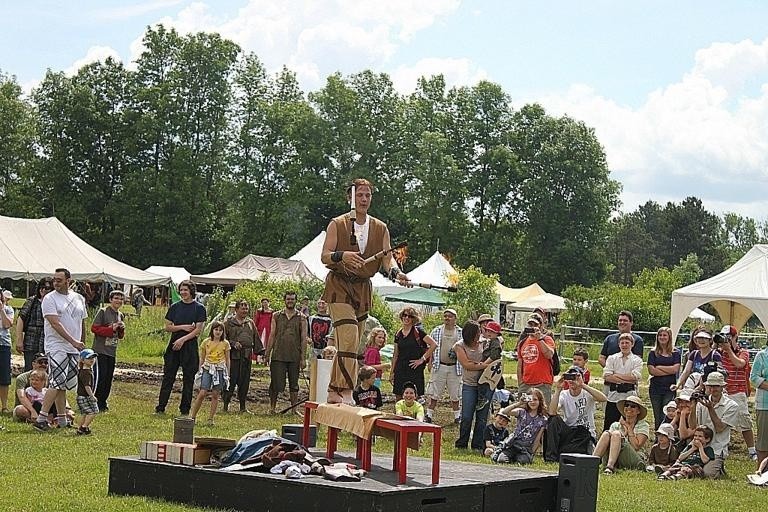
132,294,141,307
519,335,560,375
720,348,751,396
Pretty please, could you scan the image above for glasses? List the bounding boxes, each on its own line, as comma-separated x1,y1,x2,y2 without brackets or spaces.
444,314,453,318
624,404,635,408
39,285,50,290
402,314,413,318
38,360,48,365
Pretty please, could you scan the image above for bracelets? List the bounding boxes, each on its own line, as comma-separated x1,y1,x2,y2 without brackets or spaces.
391,266,401,279
330,251,344,262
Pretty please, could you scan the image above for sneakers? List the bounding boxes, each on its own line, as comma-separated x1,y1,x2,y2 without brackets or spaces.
654,465,664,473
2,405,109,435
454,416,462,424
750,452,758,462
423,415,432,422
637,461,646,471
152,410,304,426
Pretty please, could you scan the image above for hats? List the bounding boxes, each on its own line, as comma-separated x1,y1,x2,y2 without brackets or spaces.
31,351,48,361
496,408,512,422
477,314,494,322
444,309,457,319
615,323,737,441
3,290,14,299
482,321,501,333
80,349,98,359
526,313,543,328
228,302,237,308
567,365,584,378
533,305,545,313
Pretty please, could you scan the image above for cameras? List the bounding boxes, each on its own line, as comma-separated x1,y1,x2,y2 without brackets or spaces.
523,394,533,401
692,389,709,400
713,333,728,344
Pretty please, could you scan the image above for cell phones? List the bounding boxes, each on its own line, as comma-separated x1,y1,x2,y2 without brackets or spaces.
525,327,535,333
563,374,576,380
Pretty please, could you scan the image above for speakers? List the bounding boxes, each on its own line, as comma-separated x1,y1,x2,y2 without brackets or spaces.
554,451,601,511
281,423,317,448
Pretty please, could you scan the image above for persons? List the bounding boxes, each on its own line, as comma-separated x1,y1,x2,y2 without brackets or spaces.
91,290,126,413
134,288,152,316
0,277,75,423
155,279,207,417
427,309,768,484
77,348,100,434
321,178,413,407
32,268,88,432
190,291,438,417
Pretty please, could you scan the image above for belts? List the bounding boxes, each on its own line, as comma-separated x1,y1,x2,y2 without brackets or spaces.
440,362,456,365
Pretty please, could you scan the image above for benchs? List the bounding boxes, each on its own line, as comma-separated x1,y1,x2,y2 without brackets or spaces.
302,398,442,483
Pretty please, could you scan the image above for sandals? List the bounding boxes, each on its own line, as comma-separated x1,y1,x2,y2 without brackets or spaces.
666,473,678,480
657,473,667,481
603,466,614,476
